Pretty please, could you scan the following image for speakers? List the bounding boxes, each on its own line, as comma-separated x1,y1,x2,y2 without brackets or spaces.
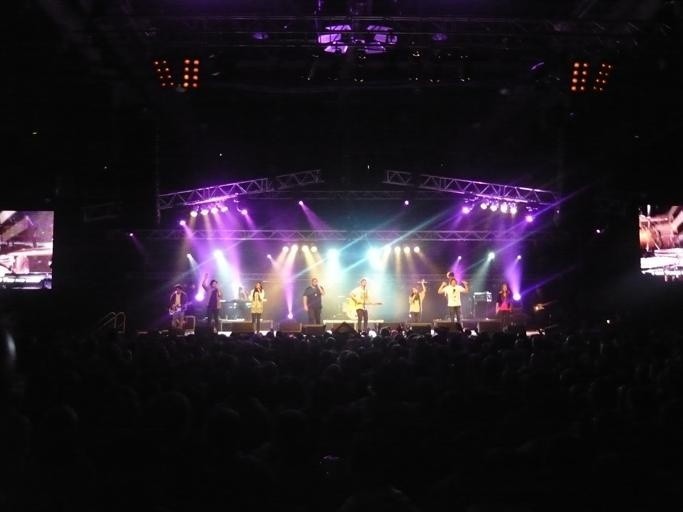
333,322,360,336
231,322,254,336
124,125,164,227
433,321,459,332
301,324,326,334
410,323,431,334
378,322,402,336
195,319,215,336
478,319,503,334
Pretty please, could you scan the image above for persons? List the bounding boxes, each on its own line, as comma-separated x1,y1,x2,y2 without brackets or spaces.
302,277,324,325
407,279,427,322
1,321,683,511
169,284,188,329
437,279,470,324
201,271,222,327
446,271,458,285
348,278,370,331
245,281,266,333
494,282,513,320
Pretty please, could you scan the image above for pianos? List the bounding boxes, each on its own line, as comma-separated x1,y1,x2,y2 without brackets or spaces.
220,299,244,303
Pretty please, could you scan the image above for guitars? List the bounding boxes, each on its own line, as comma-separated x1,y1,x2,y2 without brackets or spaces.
345,297,382,310
169,301,192,316
